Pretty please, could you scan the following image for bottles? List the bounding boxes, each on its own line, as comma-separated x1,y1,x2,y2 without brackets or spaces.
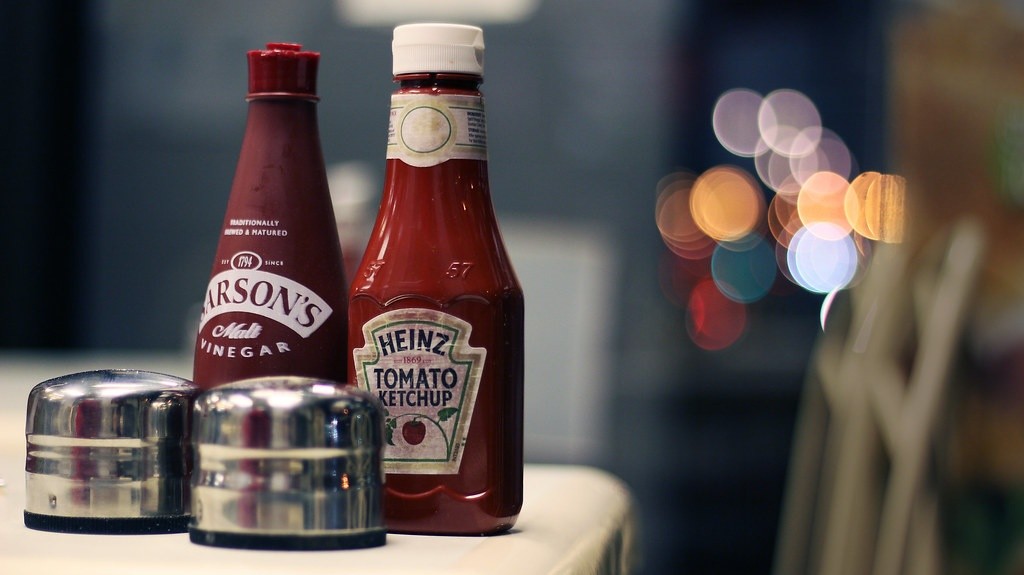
191,42,348,388
342,21,525,537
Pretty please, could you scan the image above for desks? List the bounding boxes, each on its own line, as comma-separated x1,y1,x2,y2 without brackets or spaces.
0,451,635,575
